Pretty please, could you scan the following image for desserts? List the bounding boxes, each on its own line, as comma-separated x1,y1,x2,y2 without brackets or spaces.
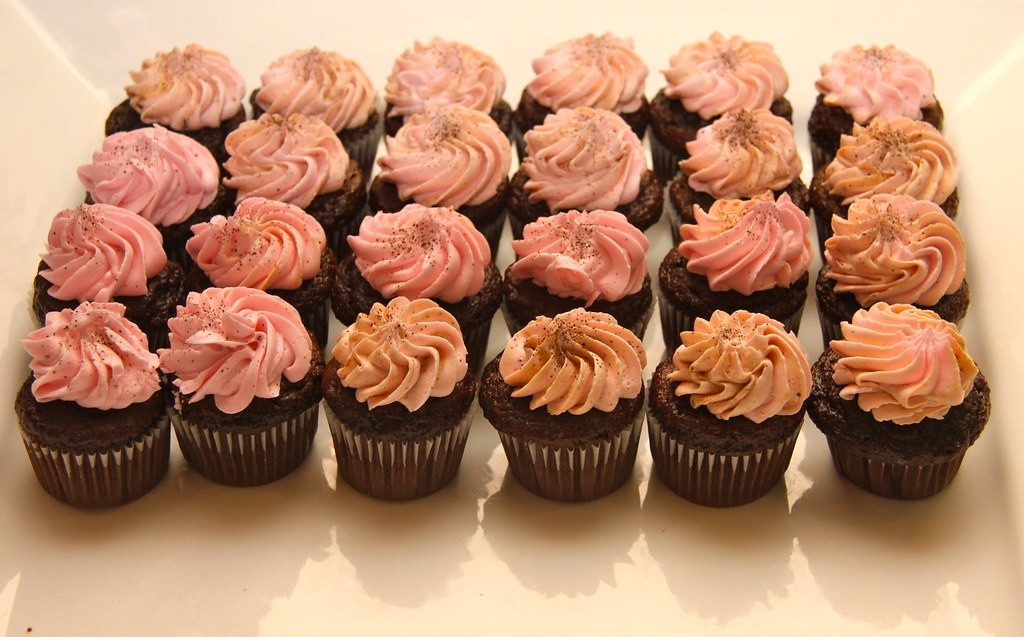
14,33,990,508
